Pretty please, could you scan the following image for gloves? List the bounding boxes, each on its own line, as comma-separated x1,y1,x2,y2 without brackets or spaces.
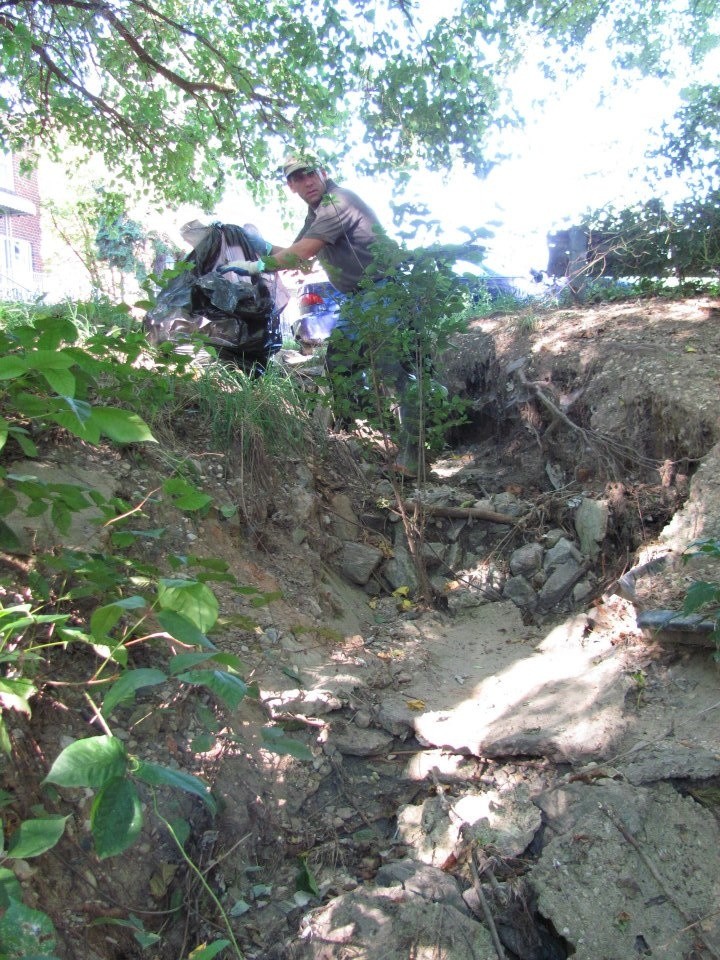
216,260,260,276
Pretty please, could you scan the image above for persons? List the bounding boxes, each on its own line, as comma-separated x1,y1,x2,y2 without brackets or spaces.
215,148,433,482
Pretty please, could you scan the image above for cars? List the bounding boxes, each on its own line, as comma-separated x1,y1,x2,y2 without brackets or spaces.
288,256,537,356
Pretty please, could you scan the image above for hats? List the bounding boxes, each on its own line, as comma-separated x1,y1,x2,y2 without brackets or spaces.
284,148,326,177
